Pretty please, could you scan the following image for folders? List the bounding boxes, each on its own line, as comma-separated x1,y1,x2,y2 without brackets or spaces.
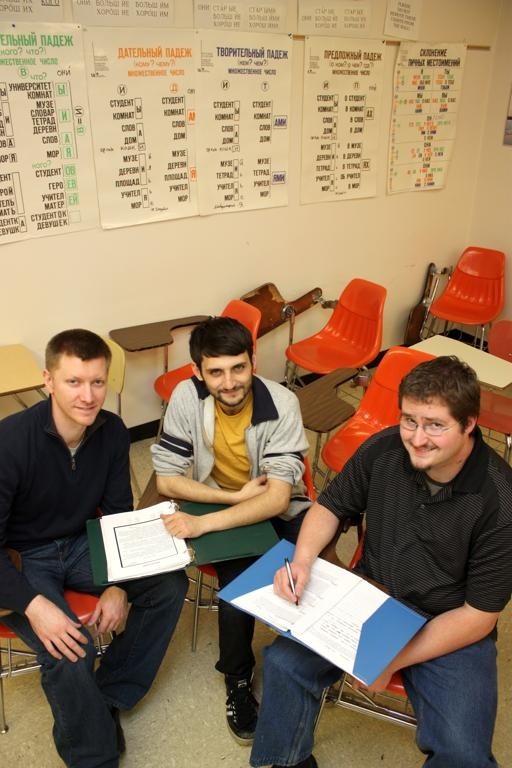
217,538,428,685
86,501,284,584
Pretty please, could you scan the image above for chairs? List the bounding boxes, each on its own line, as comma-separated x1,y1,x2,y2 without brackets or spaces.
427,246,505,350
101,334,143,499
1,590,129,734
284,277,388,392
475,320,511,466
182,456,316,651
310,514,416,744
153,298,262,446
312,343,438,498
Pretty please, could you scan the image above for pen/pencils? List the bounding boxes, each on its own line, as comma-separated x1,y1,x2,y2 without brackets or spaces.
278,556,310,609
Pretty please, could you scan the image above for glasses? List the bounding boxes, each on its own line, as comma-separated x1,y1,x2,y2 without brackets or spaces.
398,410,464,436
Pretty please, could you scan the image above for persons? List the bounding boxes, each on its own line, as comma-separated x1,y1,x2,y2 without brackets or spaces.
1,328,189,760
149,316,328,741
248,356,508,763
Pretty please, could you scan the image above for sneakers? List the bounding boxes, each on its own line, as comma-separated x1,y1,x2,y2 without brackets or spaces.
225,670,259,745
274,754,318,768
110,704,126,753
